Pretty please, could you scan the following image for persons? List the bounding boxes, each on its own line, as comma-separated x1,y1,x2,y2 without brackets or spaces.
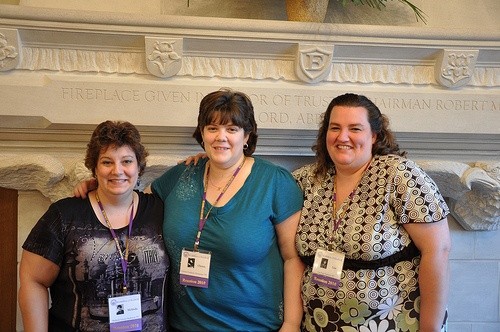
280,93,450,332
13,119,173,332
320,257,328,269
187,257,196,269
142,88,306,332
115,305,125,316
